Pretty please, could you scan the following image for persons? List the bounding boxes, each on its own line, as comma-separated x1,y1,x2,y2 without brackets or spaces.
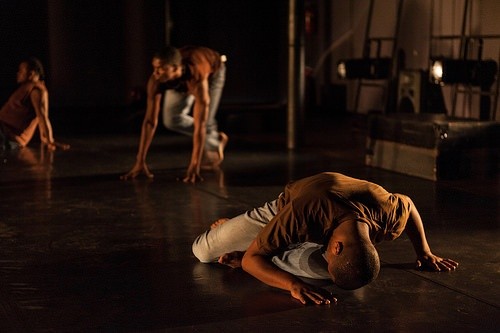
121,48,229,184
192,172,458,304
0,59,70,158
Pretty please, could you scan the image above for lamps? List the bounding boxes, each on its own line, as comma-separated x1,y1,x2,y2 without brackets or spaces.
337,39,391,80
433,37,498,90
